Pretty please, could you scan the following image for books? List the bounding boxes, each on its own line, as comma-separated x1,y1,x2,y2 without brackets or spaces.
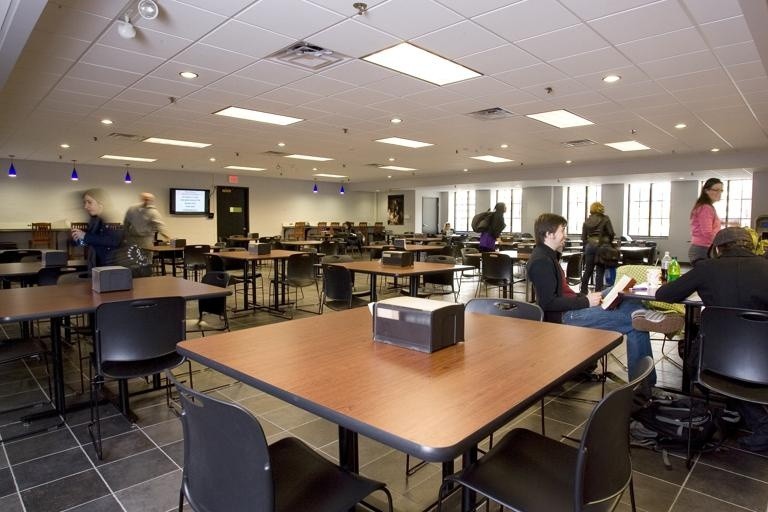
601,273,637,313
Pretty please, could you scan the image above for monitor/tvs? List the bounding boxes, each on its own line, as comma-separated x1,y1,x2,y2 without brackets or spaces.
169,186,211,215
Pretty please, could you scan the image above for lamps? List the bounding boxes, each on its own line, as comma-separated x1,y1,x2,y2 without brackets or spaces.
278,143,285,147
312,168,318,170
312,173,348,179
500,144,508,148
125,175,131,182
377,165,421,172
601,76,621,83
210,157,216,162
674,123,689,130
358,40,484,92
179,71,198,79
283,154,334,162
313,185,318,192
224,165,268,171
8,163,17,177
118,22,138,39
101,120,112,125
213,104,308,133
140,137,214,149
603,140,652,151
340,186,344,192
71,167,78,178
376,137,435,150
389,158,395,161
138,1,159,19
711,148,720,152
60,144,71,149
566,160,573,164
470,155,514,164
463,168,469,172
524,109,596,129
100,154,157,163
390,119,403,124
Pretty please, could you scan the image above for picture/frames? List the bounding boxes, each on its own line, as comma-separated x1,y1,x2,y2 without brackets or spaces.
389,196,404,225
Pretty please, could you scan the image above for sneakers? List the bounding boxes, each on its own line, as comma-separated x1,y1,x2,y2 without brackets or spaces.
633,309,683,333
737,429,768,449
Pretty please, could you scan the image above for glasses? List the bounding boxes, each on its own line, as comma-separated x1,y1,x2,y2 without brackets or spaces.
710,188,723,193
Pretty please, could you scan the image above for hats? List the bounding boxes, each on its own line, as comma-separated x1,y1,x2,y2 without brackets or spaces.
707,228,753,260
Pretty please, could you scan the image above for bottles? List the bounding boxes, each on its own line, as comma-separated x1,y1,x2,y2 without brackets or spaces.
661,251,672,282
668,256,680,282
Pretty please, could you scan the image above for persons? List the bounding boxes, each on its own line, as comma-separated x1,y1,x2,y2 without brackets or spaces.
580,202,616,294
525,213,685,407
688,178,724,268
441,221,454,237
655,227,768,455
72,187,121,274
479,202,507,254
123,191,170,260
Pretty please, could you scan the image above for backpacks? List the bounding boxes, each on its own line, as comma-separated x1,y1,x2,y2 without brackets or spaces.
629,396,741,471
472,208,494,233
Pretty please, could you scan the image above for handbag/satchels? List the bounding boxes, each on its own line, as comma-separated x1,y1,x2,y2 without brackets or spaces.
594,215,619,267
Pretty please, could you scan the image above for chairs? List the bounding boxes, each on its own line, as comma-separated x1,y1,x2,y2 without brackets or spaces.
270,242,284,251
322,254,354,264
462,298,544,323
374,222,383,234
440,246,455,255
0,336,55,412
259,237,274,243
686,305,768,469
220,248,266,308
215,242,226,250
288,222,306,240
349,221,354,227
320,240,338,255
457,233,468,242
27,222,52,250
427,241,447,247
358,222,368,245
562,254,584,286
451,241,464,257
82,297,195,460
311,221,327,237
186,272,232,336
269,252,320,320
401,255,458,304
162,370,394,512
200,254,252,318
319,263,372,318
473,252,527,300
446,355,662,512
177,244,210,279
330,222,339,234
59,271,93,284
36,268,77,287
368,242,390,260
66,222,87,261
461,247,482,286
645,242,660,267
